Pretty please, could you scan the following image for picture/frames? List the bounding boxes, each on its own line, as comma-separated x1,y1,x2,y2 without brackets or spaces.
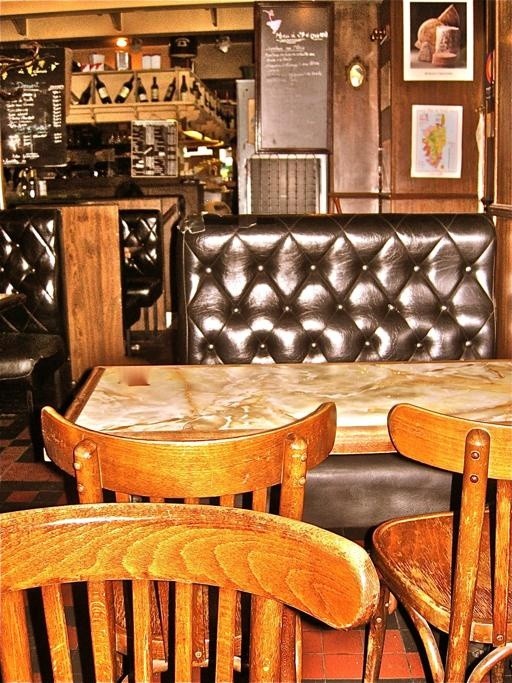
402,0,474,82
410,104,464,179
254,1,334,155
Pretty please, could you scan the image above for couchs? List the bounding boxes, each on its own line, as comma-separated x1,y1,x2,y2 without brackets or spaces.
177,214,497,527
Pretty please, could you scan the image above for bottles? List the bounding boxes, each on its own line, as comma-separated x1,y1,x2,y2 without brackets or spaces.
114,76,134,103
180,75,188,102
80,81,90,103
190,78,236,129
136,76,148,102
14,167,36,200
69,91,79,105
164,77,176,101
151,76,159,101
94,74,111,103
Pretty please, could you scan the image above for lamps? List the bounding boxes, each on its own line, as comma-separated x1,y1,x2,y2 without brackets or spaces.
215,36,231,53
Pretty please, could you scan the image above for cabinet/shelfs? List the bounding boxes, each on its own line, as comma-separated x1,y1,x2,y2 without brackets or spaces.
63,46,227,142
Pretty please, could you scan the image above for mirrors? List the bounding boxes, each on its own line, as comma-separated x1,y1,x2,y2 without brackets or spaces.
344,54,369,92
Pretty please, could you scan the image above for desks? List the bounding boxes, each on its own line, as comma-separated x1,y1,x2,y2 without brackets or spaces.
44,360,511,462
